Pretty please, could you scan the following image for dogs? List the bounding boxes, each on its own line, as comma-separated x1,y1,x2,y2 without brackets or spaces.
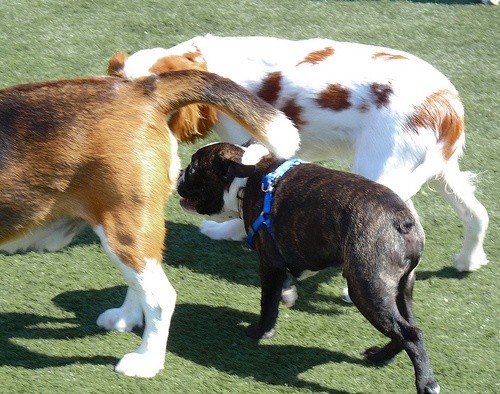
107,32,491,302
176,141,441,394
0,69,302,378
